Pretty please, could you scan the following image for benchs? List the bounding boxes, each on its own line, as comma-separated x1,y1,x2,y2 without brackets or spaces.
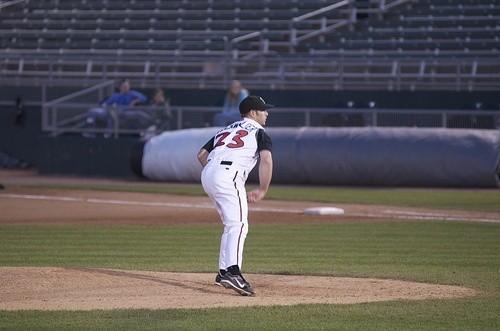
0,0,500,134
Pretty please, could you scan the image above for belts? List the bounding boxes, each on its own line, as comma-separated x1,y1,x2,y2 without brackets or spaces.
221,161,232,165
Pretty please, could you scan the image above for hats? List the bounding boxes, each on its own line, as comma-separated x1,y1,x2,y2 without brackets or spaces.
239,96,274,113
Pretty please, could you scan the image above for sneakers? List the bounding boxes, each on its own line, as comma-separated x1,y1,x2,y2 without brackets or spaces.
214,271,256,297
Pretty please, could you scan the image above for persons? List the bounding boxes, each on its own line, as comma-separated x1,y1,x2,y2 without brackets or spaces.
150,90,171,135
102,78,147,138
196,96,276,296
214,80,249,127
